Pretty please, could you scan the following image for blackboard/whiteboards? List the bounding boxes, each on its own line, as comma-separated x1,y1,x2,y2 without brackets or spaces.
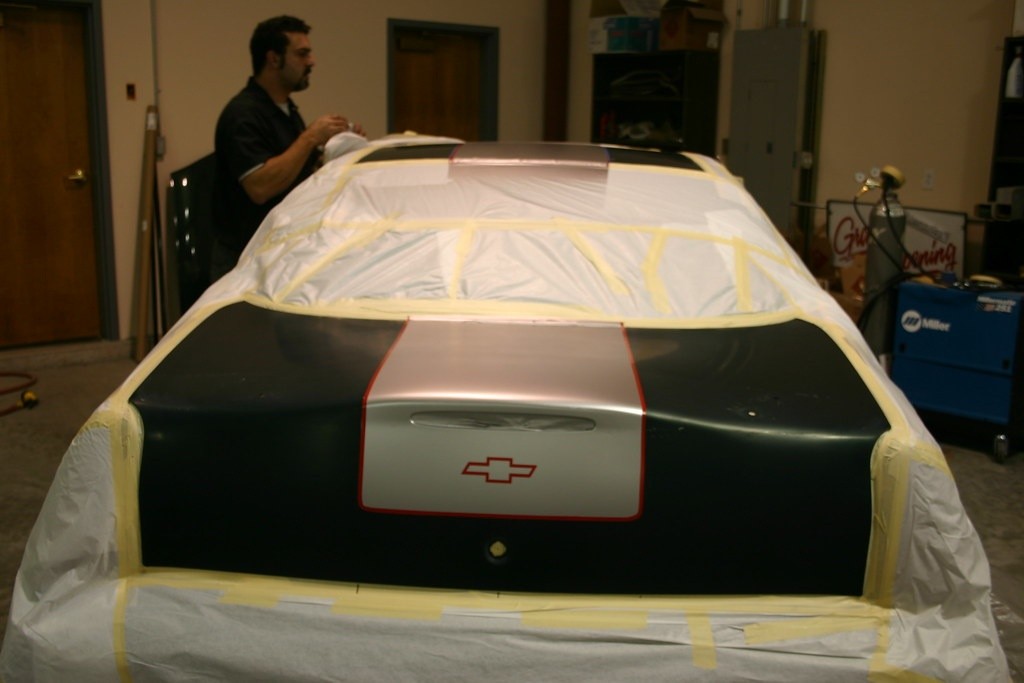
825,198,969,281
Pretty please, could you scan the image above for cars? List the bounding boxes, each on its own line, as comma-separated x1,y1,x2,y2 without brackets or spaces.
1,129,1011,683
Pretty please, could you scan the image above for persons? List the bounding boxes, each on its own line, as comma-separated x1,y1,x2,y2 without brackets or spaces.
211,14,366,281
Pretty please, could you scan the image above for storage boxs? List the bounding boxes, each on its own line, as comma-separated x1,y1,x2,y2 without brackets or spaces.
658,1,729,52
589,1,659,51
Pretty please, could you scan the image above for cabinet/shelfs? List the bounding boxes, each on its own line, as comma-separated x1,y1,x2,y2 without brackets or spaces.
591,49,718,157
